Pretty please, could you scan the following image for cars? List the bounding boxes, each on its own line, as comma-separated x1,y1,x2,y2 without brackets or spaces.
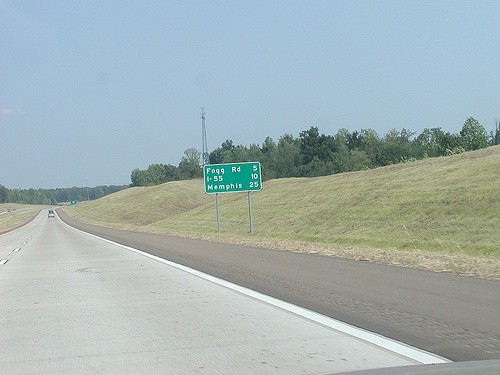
48,209,55,217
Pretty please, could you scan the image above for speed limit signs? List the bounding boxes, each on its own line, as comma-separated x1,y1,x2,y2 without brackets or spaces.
204,161,263,193
